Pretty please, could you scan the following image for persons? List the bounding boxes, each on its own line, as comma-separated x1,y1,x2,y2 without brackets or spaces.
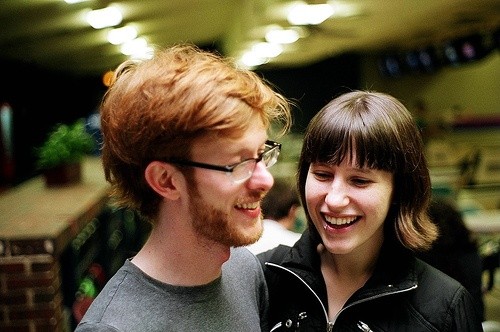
256,91,485,332
244,179,302,256
72,44,278,332
423,200,484,324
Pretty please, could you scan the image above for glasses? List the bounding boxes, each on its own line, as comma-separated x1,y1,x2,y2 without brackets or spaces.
160,139,282,183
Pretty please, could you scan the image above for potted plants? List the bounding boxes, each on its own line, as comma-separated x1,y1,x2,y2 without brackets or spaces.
41,120,96,188
